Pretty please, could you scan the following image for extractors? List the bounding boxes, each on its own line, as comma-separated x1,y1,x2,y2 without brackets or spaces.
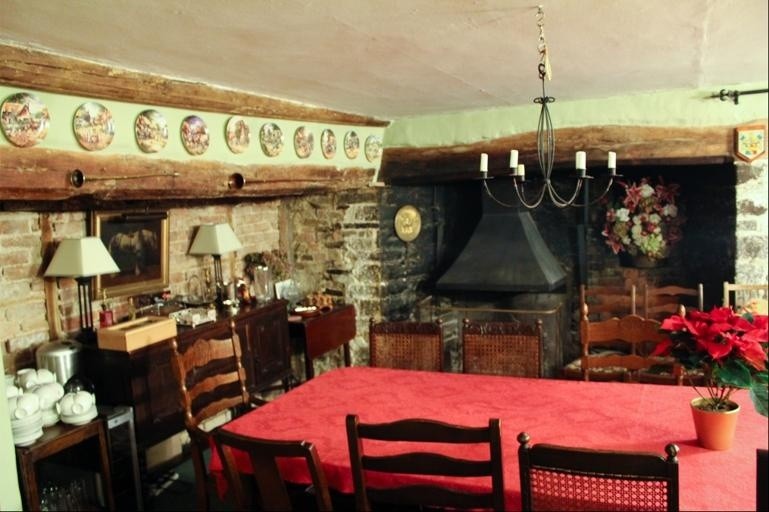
432,172,569,295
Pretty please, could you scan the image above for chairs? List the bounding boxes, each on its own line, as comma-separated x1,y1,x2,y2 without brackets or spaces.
562,282,704,386
167,319,269,512
462,318,543,378
345,414,507,512
756,448,769,511
516,431,679,511
369,317,443,372
722,281,768,312
211,427,333,512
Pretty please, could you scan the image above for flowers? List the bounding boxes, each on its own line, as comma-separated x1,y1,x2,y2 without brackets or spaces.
651,306,768,417
598,176,684,261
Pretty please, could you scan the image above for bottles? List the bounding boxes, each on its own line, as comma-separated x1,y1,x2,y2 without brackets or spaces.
99,299,115,326
282,275,306,305
128,298,135,321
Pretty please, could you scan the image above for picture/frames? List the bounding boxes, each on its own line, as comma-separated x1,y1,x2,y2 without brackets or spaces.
90,209,170,302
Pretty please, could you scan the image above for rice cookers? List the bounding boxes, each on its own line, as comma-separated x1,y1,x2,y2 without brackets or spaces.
35,340,83,384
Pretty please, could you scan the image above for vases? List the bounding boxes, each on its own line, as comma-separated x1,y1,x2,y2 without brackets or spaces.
690,398,740,450
618,254,659,269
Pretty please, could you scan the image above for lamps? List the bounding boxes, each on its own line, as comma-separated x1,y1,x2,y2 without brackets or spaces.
41,236,121,330
188,221,245,301
477,4,619,210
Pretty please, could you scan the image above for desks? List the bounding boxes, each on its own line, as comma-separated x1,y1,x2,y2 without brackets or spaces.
74,298,290,496
208,367,769,512
285,303,356,381
15,420,118,512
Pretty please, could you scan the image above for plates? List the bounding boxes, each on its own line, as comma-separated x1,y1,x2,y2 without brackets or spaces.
41,411,60,428
60,406,99,424
11,418,44,447
289,306,319,318
395,204,421,244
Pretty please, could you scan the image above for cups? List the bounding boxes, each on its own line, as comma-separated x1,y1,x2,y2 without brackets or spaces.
4,367,56,397
255,266,269,306
56,390,94,415
35,383,64,409
7,393,41,419
